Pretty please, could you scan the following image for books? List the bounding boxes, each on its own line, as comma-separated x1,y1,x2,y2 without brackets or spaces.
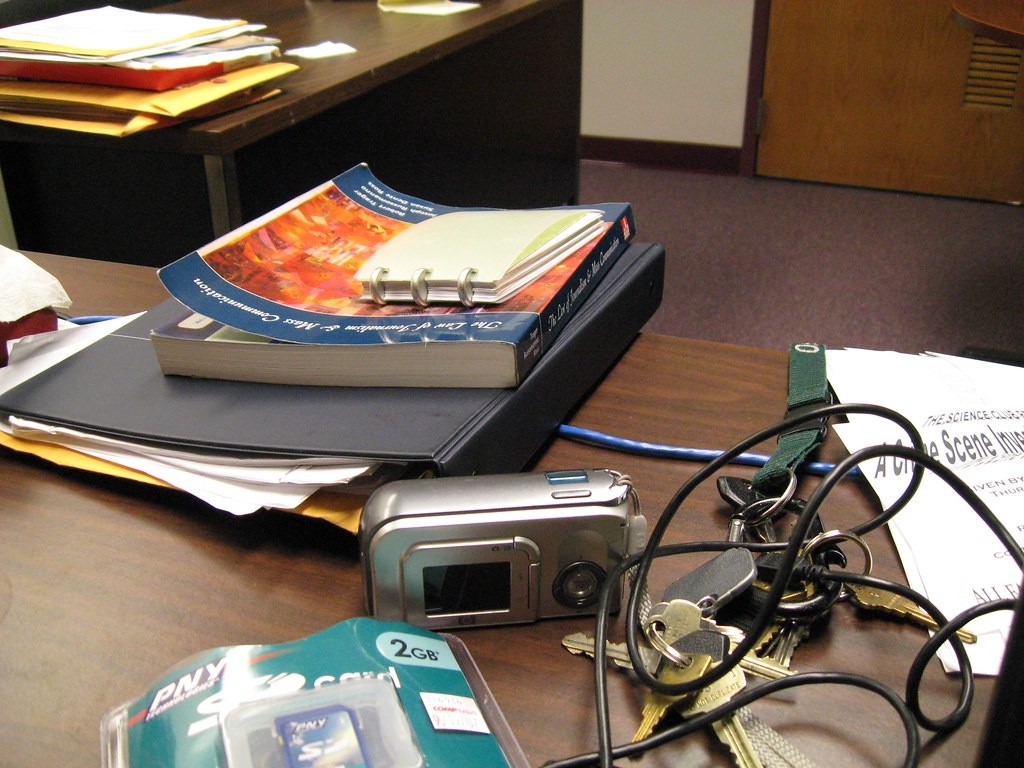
0,5,634,387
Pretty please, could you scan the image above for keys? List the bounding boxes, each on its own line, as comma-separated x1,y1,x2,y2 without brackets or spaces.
559,473,977,768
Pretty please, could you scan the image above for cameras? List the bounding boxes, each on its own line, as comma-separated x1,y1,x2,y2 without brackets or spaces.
358,470,632,630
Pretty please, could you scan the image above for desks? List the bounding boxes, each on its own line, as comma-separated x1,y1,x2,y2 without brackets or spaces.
0,249,1001,768
0,0,583,265
